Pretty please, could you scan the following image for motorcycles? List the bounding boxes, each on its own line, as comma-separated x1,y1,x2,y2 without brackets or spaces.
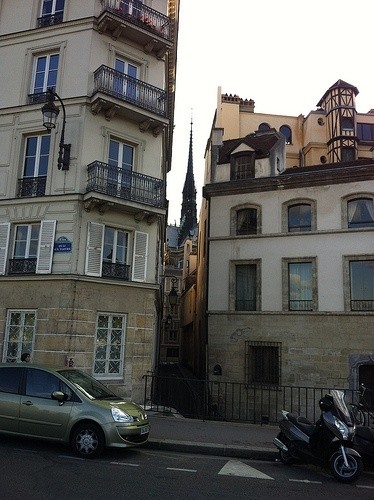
272,389,363,483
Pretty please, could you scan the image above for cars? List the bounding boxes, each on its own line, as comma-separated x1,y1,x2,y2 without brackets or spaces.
0,362,152,460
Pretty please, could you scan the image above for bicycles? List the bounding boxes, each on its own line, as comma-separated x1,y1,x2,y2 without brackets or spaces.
350,383,374,426
